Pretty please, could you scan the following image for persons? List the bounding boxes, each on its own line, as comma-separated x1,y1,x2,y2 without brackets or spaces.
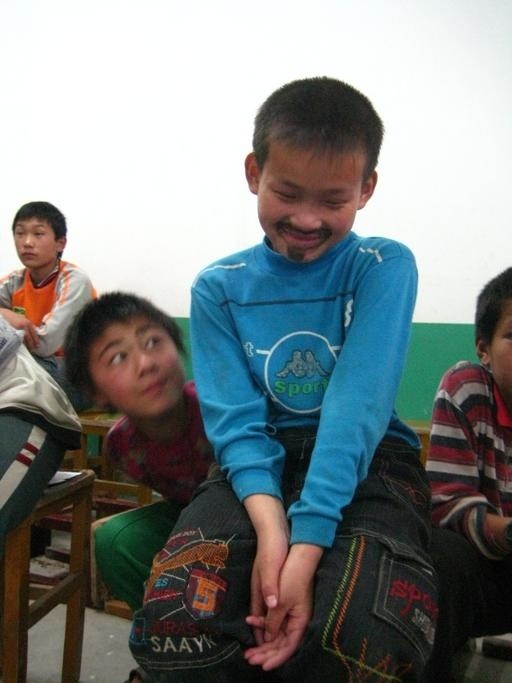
0,198,104,421
58,291,206,612
410,256,508,683
131,76,442,678
2,301,75,539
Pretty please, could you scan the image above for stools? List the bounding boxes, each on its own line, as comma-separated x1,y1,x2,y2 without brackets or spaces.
0,412,154,682
